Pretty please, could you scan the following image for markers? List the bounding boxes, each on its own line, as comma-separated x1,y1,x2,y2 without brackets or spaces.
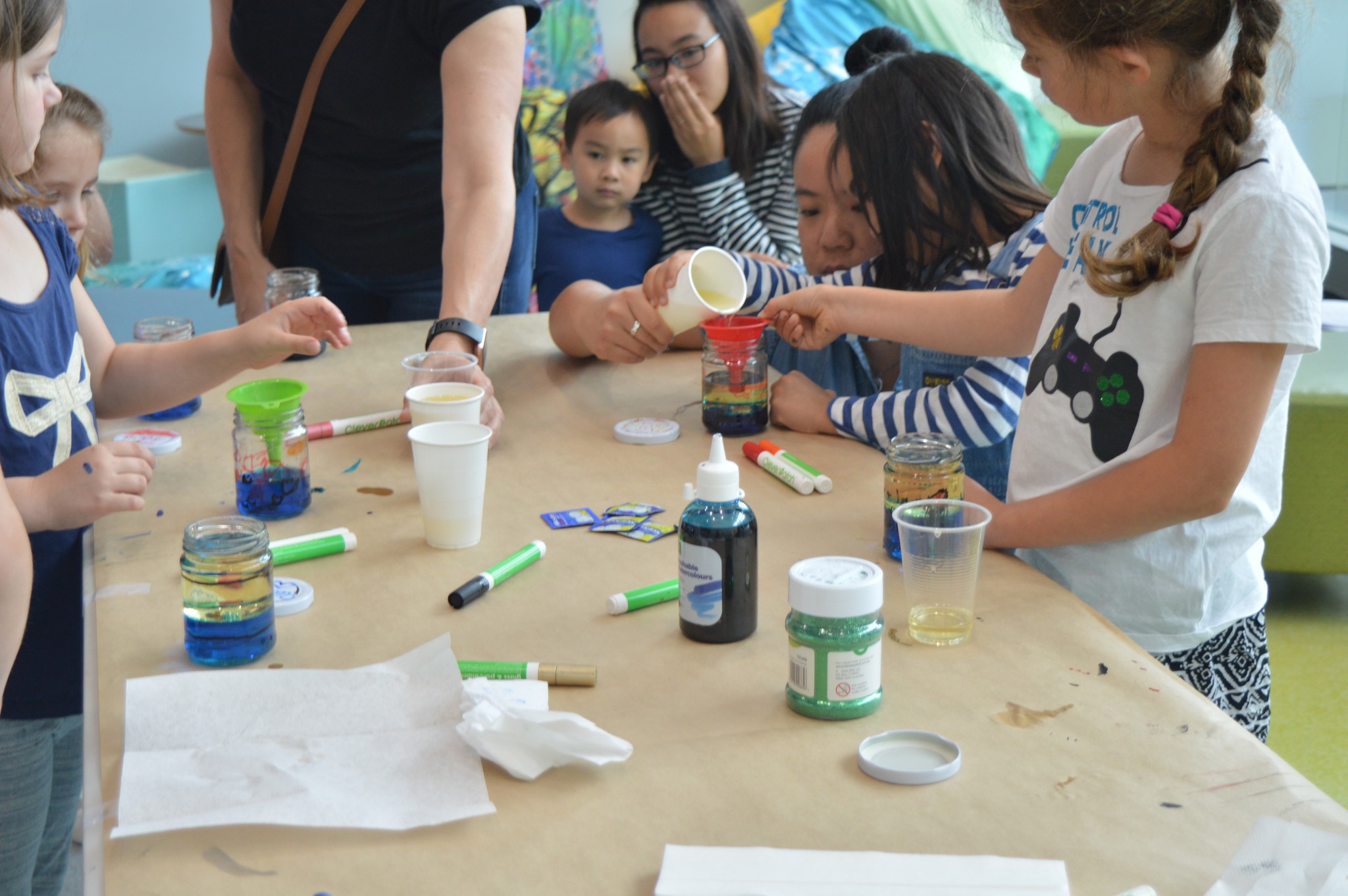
447,540,545,610
286,408,408,440
743,441,814,495
268,526,359,565
454,659,599,690
607,578,679,615
757,439,833,493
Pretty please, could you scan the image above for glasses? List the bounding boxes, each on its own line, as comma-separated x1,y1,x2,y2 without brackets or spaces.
632,29,723,79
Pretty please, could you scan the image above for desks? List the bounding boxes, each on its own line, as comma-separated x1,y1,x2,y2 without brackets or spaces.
92,313,1348,896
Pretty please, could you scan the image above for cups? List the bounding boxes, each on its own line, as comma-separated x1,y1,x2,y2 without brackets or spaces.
400,352,478,386
893,499,992,645
407,421,493,549
655,246,748,336
406,381,485,424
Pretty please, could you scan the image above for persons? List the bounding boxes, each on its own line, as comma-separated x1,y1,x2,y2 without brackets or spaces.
203,0,550,448
757,2,1330,755
1,0,1330,895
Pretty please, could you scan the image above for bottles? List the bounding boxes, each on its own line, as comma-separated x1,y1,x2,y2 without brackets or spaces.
262,266,327,361
233,402,311,520
785,555,884,719
180,516,278,667
883,432,966,561
134,316,201,421
701,333,769,437
679,430,758,644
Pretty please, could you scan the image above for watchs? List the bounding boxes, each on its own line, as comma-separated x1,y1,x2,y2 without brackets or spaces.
426,316,489,373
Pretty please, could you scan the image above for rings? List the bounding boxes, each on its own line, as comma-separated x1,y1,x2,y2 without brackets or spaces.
677,119,686,126
630,320,640,338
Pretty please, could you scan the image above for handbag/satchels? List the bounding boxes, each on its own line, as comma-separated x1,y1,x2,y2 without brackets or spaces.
211,222,236,305
